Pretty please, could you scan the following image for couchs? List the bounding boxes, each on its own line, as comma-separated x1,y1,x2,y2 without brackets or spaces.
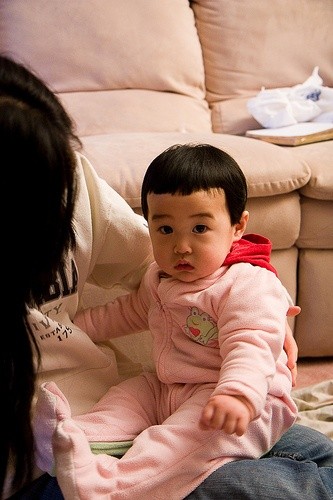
0,0,333,358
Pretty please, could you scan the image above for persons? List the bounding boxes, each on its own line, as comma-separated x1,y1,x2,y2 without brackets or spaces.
30,142,299,500
0,50,333,500
